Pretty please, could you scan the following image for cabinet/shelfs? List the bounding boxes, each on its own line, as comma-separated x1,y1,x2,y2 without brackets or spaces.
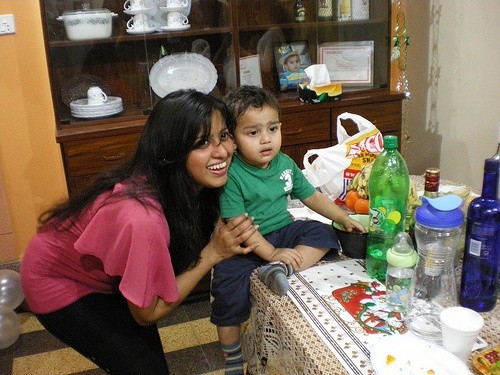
39,0,406,204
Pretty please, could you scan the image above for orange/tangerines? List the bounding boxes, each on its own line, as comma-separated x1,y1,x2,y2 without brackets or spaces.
344,191,369,215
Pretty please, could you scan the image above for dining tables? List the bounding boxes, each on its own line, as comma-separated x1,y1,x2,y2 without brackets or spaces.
243,175,499,375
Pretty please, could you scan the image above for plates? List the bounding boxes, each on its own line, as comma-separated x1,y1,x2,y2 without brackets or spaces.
70,96,126,120
123,7,191,34
370,335,472,375
472,345,500,375
149,52,218,99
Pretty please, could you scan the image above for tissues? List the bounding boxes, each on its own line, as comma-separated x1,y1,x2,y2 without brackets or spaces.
298,63,342,106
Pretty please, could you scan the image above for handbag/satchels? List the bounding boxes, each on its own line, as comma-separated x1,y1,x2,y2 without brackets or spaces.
302,111,385,215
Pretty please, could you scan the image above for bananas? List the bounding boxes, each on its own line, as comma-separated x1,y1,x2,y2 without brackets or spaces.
349,163,417,208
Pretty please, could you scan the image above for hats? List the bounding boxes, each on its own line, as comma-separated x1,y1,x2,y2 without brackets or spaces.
278,44,299,65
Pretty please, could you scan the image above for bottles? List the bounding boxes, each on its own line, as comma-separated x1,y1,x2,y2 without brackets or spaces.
365,134,499,341
295,0,370,22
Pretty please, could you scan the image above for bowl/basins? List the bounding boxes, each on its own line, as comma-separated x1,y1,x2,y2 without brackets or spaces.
57,3,118,40
332,214,370,259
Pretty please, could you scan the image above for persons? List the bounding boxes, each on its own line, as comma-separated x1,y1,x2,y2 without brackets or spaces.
279,48,309,91
204,84,367,375
21,87,260,375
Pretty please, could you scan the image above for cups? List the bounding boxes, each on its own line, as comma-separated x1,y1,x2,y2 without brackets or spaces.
88,85,107,105
440,306,485,364
123,0,188,29
